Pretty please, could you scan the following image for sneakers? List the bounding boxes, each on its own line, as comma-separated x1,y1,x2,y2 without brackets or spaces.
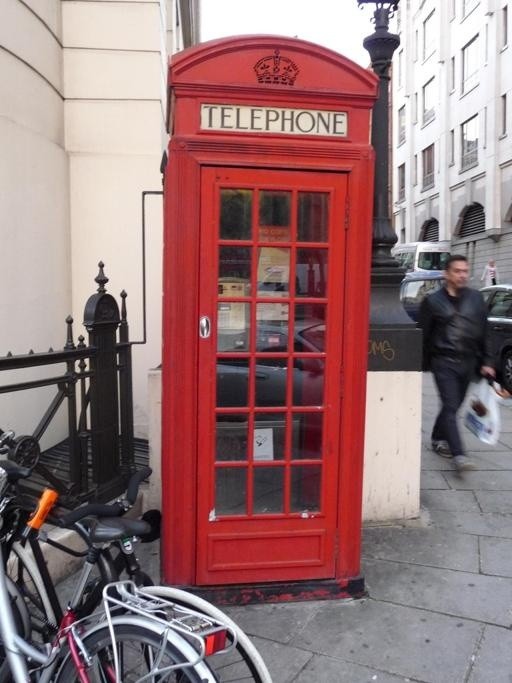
431,437,456,460
452,455,474,476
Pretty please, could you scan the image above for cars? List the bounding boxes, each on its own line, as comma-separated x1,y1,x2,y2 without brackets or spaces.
401,272,447,319
477,284,512,396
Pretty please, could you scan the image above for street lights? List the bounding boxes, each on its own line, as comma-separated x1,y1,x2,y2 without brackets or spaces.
354,0,422,524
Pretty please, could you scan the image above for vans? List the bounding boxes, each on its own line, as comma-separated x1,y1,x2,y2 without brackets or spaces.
392,240,452,274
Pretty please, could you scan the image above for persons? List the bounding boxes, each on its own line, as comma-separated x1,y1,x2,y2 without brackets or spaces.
416,254,495,471
480,260,499,286
